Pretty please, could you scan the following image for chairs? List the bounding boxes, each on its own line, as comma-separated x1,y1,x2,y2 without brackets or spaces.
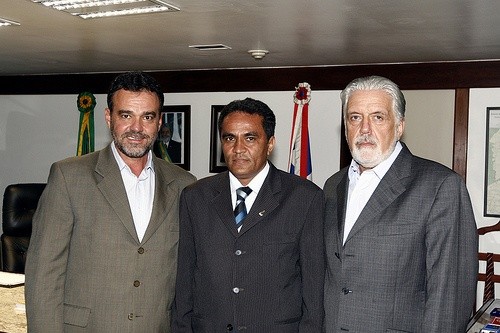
0,183,46,274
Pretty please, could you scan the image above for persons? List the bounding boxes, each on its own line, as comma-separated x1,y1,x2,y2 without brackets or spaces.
153,120,181,164
24,71,197,333
323,76,479,333
169,98,327,333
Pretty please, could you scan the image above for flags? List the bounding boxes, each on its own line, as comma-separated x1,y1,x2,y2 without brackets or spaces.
75,92,96,156
286,82,313,181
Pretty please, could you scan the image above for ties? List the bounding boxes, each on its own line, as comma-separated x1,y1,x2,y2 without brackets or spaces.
234,187,253,233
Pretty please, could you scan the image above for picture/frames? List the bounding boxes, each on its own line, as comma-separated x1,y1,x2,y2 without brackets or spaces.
153,104,191,171
208,105,228,173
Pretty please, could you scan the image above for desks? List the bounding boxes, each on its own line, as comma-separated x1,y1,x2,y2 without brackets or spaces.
0,271,27,333
